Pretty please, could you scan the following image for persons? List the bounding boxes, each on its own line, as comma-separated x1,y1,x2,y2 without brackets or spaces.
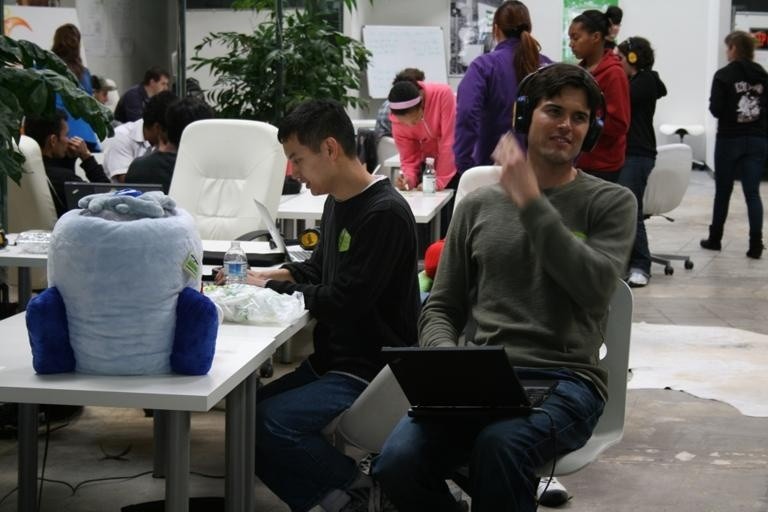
25,22,215,194
700,31,768,258
451,0,554,177
388,68,461,191
214,99,423,512
569,17,633,184
616,37,666,287
582,10,617,49
373,63,637,512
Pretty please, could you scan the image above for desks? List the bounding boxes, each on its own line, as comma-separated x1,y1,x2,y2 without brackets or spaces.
0,283,309,512
0,231,283,315
276,190,454,258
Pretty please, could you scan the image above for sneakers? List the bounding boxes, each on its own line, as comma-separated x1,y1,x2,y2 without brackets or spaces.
536,476,570,509
626,267,650,287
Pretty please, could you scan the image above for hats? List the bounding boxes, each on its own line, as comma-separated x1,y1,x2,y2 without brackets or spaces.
93,73,119,93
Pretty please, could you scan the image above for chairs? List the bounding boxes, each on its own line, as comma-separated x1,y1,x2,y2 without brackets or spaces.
536,265,631,477
168,119,288,241
320,365,413,512
420,165,506,291
642,143,693,276
374,137,399,190
0,133,58,290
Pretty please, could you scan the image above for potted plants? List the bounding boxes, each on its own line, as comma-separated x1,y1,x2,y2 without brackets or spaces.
186,0,375,194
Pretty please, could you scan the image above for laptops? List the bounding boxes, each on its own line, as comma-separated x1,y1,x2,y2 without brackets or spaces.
381,344,560,419
253,197,314,262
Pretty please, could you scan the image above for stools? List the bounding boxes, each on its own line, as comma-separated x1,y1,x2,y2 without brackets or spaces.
660,124,705,170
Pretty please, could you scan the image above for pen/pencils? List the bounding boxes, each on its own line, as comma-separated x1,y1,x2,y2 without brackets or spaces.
398,169,410,190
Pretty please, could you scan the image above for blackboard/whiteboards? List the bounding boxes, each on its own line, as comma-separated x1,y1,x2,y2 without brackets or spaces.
362,25,448,100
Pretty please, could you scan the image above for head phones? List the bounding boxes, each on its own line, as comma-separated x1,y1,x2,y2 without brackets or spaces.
301,225,321,249
626,37,638,65
512,62,608,154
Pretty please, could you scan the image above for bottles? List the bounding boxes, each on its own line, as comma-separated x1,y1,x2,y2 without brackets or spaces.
423,156,438,194
224,242,246,285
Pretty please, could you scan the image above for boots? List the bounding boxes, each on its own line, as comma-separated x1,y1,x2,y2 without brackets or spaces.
746,233,765,259
700,224,723,250
